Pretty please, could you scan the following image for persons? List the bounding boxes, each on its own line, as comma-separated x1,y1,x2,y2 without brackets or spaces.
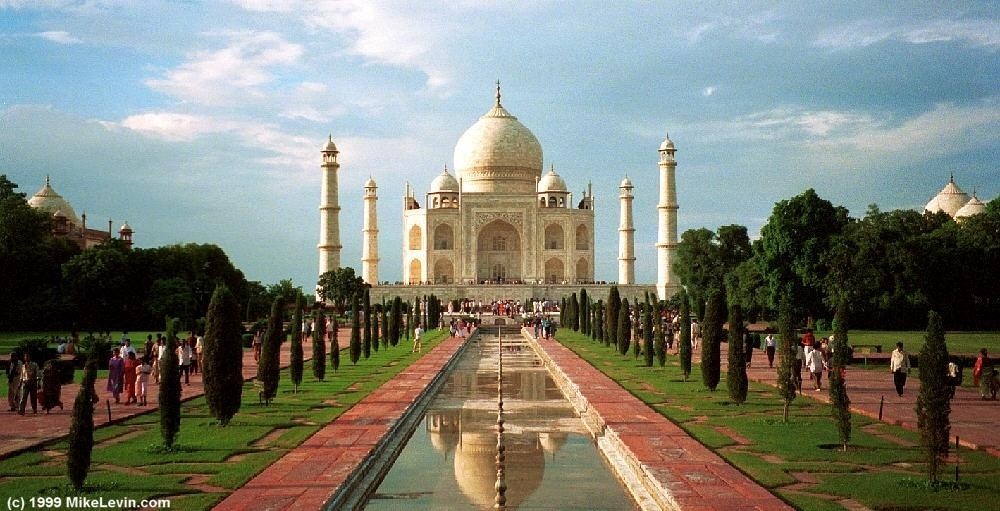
974,348,993,398
657,306,834,392
7,310,350,417
356,275,659,353
946,356,961,399
890,341,911,397
